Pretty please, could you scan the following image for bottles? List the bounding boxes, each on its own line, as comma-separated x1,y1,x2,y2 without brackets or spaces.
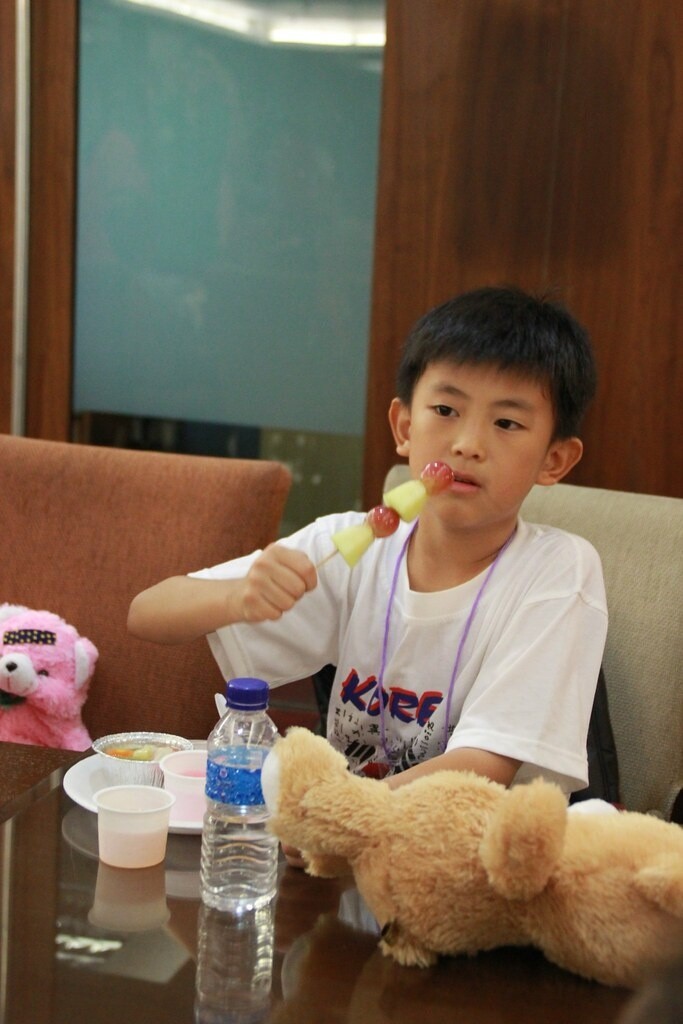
197,676,282,914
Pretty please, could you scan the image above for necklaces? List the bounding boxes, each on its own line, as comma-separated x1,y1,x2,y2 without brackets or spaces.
374,523,518,758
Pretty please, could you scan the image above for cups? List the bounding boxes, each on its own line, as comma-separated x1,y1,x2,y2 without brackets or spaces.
158,749,208,826
91,784,175,869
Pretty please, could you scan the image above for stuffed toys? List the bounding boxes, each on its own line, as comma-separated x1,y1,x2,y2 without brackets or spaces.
0,603,97,751
259,727,683,1024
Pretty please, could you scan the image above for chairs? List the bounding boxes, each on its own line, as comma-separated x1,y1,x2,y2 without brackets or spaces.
383,466,683,820
1,431,293,741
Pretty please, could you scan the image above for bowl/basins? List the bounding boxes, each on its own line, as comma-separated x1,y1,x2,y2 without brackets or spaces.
90,730,194,787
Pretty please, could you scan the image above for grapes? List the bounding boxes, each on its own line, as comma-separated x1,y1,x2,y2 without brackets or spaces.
365,505,400,538
420,460,454,495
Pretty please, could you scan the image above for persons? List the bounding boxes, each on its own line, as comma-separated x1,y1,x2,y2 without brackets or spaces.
128,285,610,806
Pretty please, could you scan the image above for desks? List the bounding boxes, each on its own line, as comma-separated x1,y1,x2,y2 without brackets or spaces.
0,740,641,1024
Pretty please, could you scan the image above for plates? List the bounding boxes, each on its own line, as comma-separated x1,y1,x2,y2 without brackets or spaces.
62,739,209,835
